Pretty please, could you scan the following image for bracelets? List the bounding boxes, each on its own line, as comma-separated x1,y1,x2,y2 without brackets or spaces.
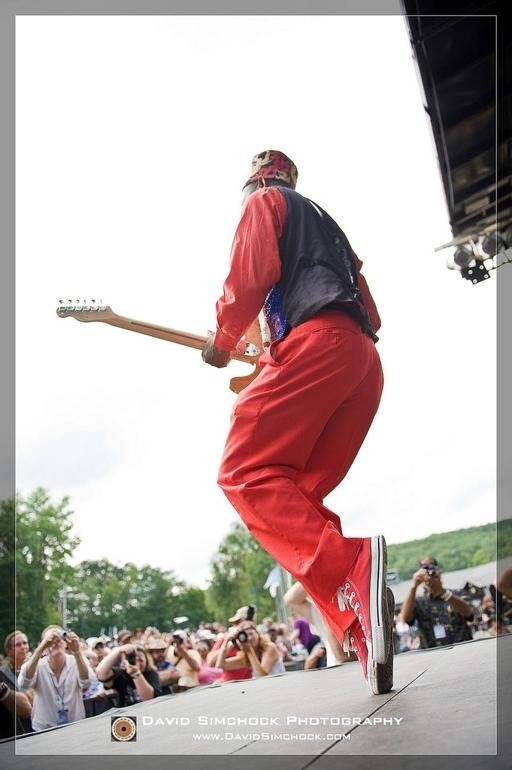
440,589,453,603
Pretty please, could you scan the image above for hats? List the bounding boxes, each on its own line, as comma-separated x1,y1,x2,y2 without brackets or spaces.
117,629,133,644
242,149,299,191
90,637,104,649
228,604,257,624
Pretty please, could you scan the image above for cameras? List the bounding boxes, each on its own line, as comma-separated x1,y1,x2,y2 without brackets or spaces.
237,631,247,643
56,631,68,640
425,569,435,576
173,635,183,645
128,651,135,665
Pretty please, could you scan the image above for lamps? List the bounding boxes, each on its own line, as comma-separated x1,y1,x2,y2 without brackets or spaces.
438,218,510,281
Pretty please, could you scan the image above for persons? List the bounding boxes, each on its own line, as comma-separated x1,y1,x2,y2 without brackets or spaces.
200,150,395,694
400,556,475,650
0,581,512,738
494,565,512,601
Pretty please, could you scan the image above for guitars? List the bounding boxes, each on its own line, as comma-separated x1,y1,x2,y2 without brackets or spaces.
56,300,264,394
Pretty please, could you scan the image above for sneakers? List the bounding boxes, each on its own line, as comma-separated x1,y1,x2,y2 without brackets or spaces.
344,584,396,698
338,534,391,663
